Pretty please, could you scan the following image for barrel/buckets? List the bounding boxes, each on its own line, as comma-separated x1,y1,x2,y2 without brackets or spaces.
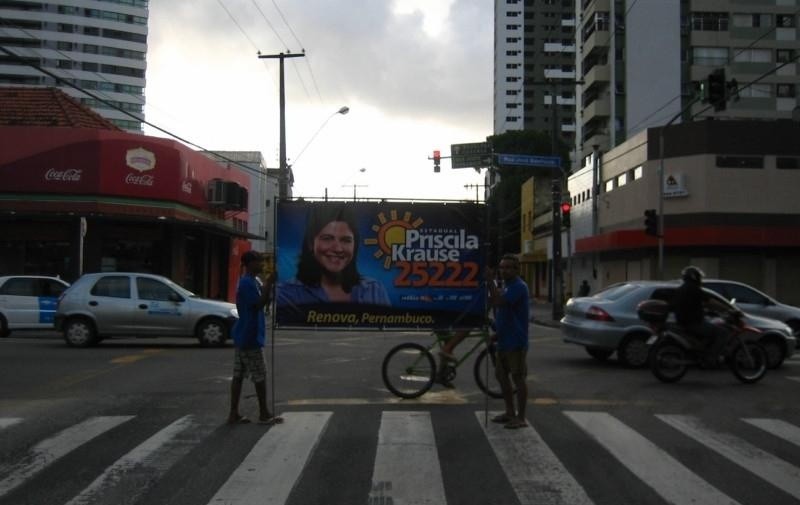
491,415,527,428
227,417,247,422
257,416,279,424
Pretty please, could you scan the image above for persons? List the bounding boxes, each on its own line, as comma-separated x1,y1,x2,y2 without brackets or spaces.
486,251,530,431
668,265,747,368
432,316,492,390
577,279,590,296
276,208,392,306
224,249,285,427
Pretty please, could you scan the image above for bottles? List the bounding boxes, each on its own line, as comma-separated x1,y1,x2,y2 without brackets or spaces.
241,251,264,263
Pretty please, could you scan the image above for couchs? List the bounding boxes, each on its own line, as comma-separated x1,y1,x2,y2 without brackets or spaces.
707,68,728,110
433,150,441,173
645,209,658,239
562,203,570,228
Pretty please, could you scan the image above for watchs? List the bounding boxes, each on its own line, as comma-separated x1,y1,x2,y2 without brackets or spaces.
634,295,770,387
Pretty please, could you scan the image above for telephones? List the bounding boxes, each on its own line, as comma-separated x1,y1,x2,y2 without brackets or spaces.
497,154,561,168
451,143,493,154
452,155,492,169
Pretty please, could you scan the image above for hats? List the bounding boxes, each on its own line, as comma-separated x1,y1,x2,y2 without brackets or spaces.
0,273,73,338
679,278,798,337
55,270,242,351
563,278,798,372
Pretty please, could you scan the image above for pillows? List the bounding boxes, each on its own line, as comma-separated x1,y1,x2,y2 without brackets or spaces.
681,266,703,287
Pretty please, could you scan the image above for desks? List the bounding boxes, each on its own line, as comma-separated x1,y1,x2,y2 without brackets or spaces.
278,107,349,198
340,168,368,196
